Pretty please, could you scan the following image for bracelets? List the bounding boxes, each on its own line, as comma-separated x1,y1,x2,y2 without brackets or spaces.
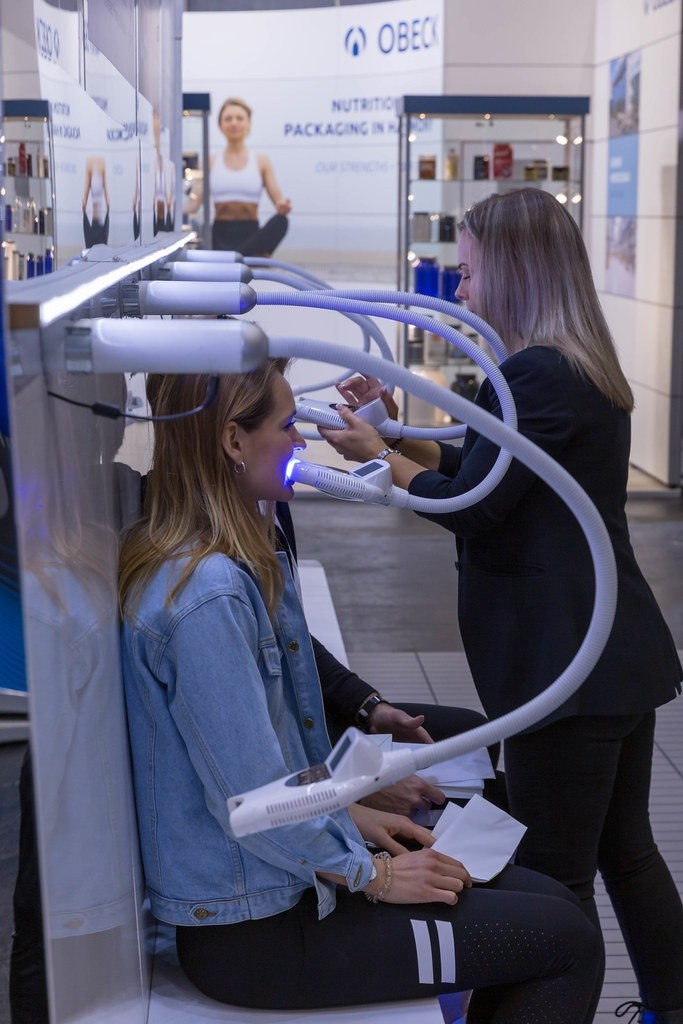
365,851,393,904
359,695,391,732
377,449,402,460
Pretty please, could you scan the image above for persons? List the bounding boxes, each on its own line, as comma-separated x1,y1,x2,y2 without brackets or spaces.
82,106,176,250
318,188,683,1024
183,98,292,260
118,357,606,1024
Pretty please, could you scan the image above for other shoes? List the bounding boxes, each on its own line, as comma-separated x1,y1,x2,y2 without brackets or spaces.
615,999,678,1023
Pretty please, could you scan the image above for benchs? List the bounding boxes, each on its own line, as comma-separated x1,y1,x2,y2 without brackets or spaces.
144,560,447,1024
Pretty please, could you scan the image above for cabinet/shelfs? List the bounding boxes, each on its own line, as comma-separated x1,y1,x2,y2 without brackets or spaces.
394,96,591,426
